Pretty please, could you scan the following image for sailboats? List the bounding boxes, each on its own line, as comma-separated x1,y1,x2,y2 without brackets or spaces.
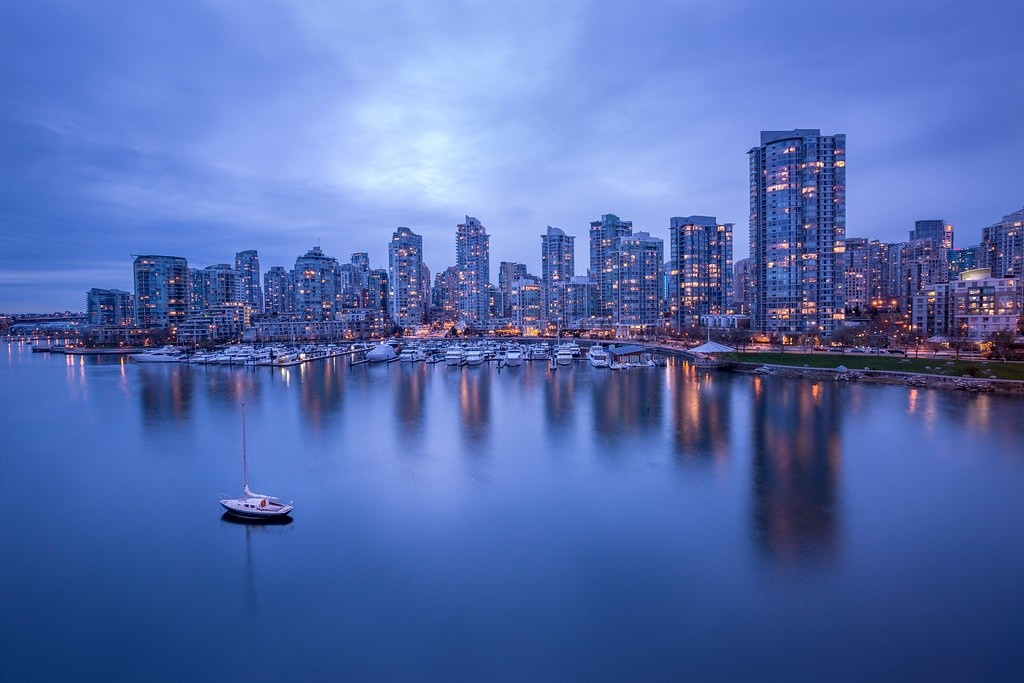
217,400,294,521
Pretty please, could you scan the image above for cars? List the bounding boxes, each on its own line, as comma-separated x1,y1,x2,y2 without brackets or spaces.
813,347,843,351
870,346,885,354
851,347,865,353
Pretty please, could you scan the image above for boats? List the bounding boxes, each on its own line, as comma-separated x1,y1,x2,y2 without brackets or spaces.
26,339,33,344
178,339,608,367
126,345,188,363
